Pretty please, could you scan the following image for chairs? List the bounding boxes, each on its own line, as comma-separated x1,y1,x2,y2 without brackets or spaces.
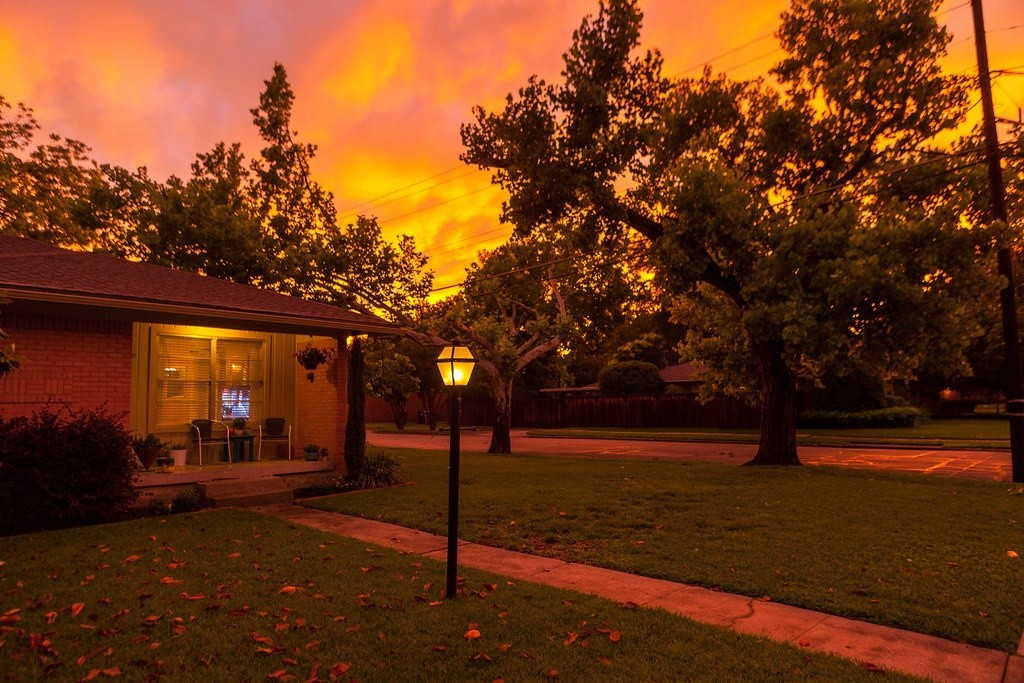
189,419,231,466
258,417,292,461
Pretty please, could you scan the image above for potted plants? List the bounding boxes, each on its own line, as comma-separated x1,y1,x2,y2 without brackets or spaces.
132,433,172,472
163,459,175,473
156,441,173,470
293,340,336,371
168,439,187,467
303,444,320,461
232,417,248,435
322,447,328,461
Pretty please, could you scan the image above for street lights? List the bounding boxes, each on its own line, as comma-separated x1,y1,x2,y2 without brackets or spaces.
437,344,478,596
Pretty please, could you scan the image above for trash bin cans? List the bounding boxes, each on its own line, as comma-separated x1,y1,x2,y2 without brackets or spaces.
417,410,425,424
424,411,431,425
399,411,408,424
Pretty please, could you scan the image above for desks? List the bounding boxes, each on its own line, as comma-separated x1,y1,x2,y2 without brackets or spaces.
222,434,257,463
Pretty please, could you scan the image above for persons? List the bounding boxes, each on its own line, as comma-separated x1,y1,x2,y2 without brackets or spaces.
231,390,246,415
